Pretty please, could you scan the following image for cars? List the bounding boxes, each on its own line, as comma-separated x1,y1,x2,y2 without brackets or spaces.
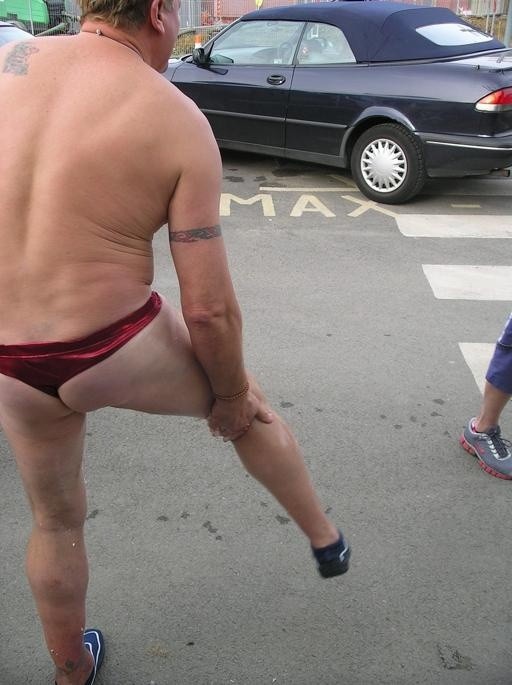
160,2,511,203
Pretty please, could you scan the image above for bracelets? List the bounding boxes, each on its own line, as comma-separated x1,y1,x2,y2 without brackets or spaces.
214,382,249,401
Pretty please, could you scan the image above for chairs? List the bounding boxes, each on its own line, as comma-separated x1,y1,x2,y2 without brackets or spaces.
289,40,321,64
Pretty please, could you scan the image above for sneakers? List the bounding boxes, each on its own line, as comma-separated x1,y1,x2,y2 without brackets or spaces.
460,416,512,479
311,530,351,579
54,629,104,685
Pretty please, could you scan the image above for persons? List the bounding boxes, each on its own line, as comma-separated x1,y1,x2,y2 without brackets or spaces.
458,312,512,481
0,0,353,685
